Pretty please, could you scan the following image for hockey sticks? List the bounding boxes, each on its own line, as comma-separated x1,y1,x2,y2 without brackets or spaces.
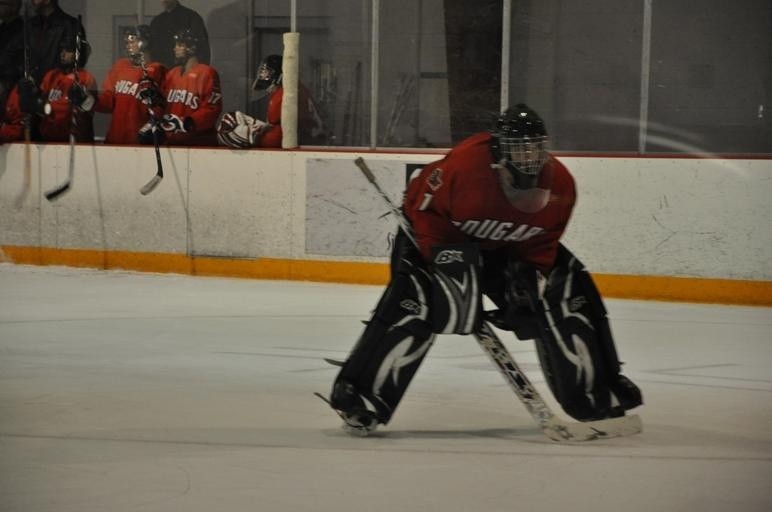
355,154,643,442
7,3,36,212
43,14,81,199
133,27,163,195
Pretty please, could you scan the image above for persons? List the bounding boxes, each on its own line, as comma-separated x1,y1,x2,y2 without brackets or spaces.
330,103,644,437
0,0,88,87
0,0,24,48
149,1,211,65
70,24,168,145
216,56,330,148
1,55,38,142
138,29,222,146
18,38,96,144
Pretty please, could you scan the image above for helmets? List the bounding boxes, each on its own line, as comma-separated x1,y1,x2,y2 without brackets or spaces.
173,28,211,66
252,54,282,91
58,37,91,67
492,103,550,187
124,24,154,58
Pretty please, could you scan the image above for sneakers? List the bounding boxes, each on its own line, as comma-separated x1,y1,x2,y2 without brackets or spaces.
330,381,380,438
